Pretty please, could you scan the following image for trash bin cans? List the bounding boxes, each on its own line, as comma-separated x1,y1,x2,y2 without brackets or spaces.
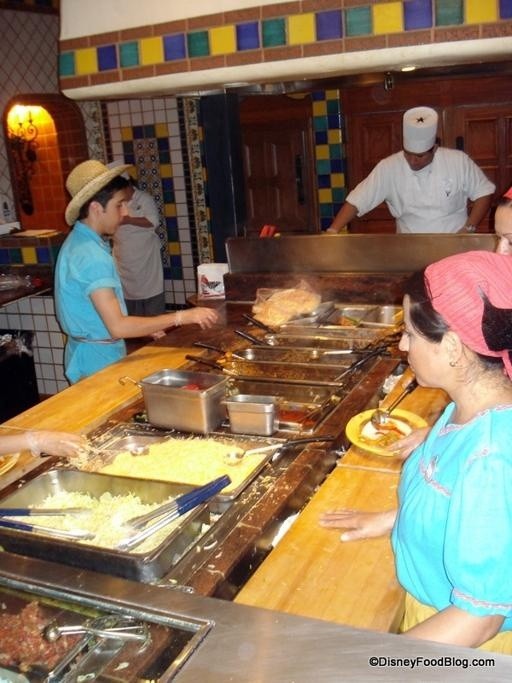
0,328,38,425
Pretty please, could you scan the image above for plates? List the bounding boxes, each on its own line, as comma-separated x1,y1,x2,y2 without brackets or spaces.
0,452,20,476
345,407,428,457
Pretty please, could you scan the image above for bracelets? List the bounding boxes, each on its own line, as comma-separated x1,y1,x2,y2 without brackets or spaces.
175,310,184,328
464,224,477,232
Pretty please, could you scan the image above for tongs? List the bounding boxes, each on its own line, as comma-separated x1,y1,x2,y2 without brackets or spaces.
111,474,233,552
0,507,97,540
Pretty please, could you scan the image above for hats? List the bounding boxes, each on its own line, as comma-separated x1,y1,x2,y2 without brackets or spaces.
65,160,132,226
403,107,438,154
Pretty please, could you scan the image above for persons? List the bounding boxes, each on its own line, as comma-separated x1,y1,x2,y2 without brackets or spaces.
315,247,511,657
0,431,91,459
494,185,512,256
320,144,498,236
102,159,167,315
53,157,221,386
